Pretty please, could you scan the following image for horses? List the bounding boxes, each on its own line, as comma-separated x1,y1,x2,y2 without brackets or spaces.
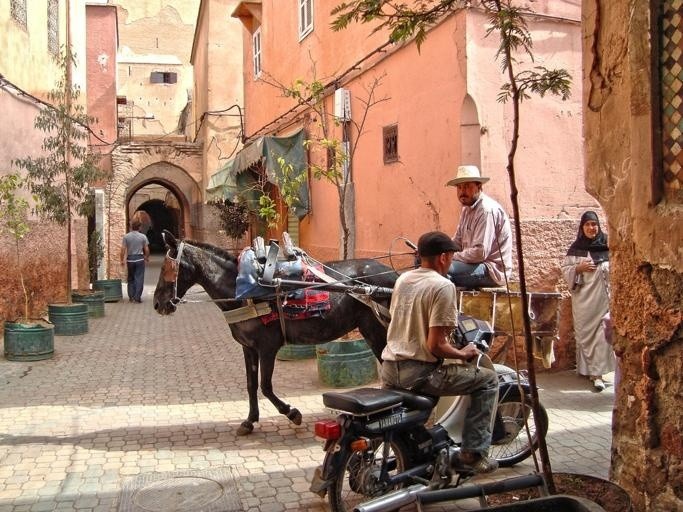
152,230,400,436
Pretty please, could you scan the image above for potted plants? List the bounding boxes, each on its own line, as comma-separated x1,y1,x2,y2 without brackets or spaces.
249,53,392,388
0,37,121,364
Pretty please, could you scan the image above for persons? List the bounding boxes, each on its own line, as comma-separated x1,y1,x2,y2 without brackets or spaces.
560,211,615,390
412,164,513,288
377,230,500,476
120,221,151,303
146,226,155,243
159,225,166,255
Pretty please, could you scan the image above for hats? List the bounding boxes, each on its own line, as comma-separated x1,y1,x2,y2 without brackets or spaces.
418,231,460,255
446,165,490,187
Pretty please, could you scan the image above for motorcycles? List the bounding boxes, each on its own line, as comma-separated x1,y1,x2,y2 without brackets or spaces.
309,316,548,512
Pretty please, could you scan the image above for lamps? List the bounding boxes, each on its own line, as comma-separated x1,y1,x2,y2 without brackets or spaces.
119,112,155,122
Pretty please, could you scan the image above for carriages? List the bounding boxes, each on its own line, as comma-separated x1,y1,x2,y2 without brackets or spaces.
153,231,561,436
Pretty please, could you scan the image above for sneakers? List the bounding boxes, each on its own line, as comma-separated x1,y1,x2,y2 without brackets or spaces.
129,297,143,303
454,452,498,475
590,373,604,390
